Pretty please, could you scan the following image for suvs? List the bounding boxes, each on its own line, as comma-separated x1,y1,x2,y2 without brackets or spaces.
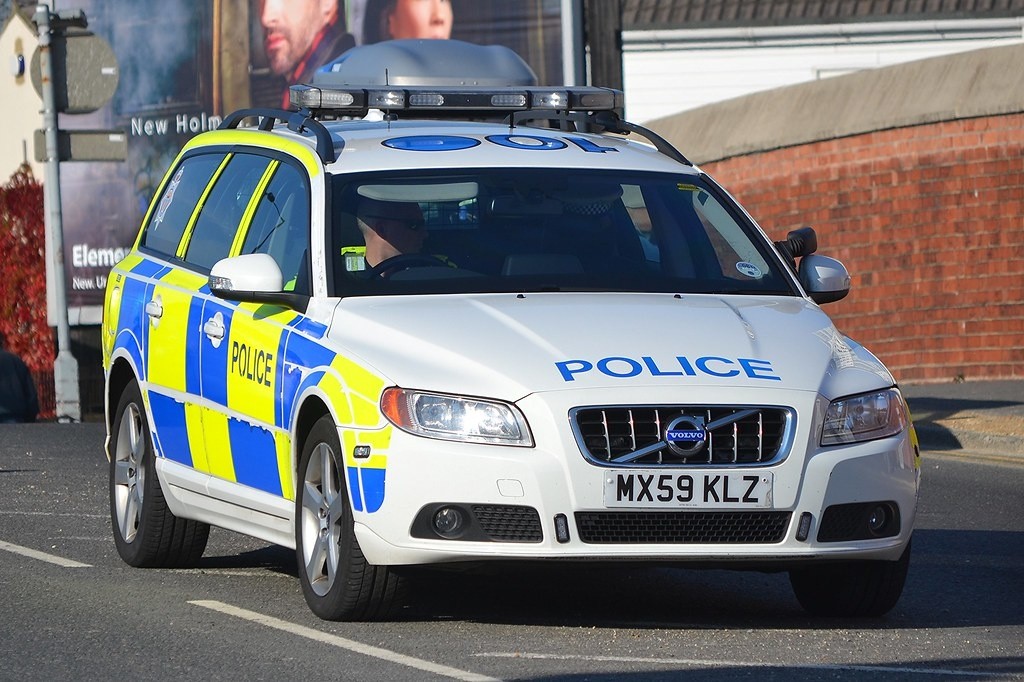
99,38,923,624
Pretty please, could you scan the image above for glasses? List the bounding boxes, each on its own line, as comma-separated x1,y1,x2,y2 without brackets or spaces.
365,215,426,230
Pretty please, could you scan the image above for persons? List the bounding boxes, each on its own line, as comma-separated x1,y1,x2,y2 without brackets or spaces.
257,0,355,124
282,198,458,291
361,0,454,46
